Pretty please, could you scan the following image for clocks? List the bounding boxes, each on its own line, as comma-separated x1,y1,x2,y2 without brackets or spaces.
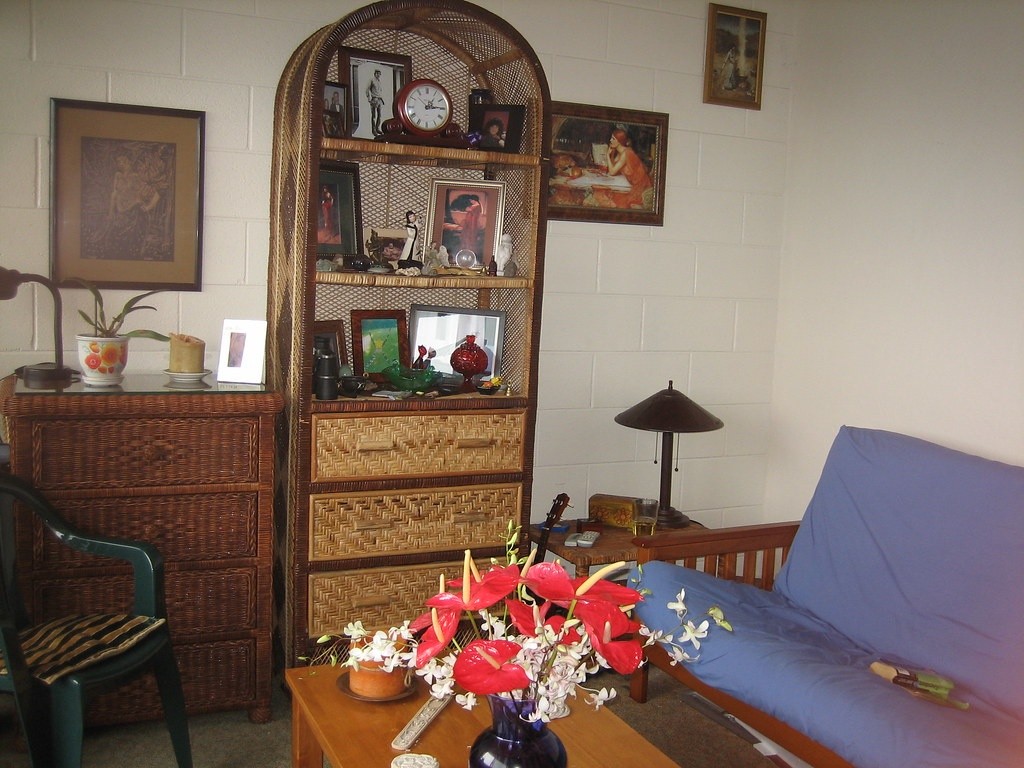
392,80,453,135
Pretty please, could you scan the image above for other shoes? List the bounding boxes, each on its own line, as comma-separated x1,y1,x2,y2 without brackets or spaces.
376,129,382,134
372,130,377,136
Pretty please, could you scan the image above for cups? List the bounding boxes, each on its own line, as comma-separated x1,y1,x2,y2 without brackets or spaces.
350,630,409,698
631,498,658,536
339,376,365,398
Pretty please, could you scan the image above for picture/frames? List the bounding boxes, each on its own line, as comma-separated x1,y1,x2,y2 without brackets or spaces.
314,320,348,372
321,109,347,138
338,46,412,142
524,99,668,227
324,81,349,135
469,104,525,153
423,177,507,274
702,3,767,110
46,97,206,292
316,158,364,265
362,228,408,269
349,309,411,382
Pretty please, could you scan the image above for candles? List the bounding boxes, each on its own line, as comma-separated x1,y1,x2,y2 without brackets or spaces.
168,333,206,373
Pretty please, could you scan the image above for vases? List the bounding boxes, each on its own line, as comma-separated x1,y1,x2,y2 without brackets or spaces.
451,335,488,392
412,356,423,369
422,360,430,369
468,690,567,768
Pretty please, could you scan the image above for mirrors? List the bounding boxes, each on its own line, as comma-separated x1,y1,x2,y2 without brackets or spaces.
409,303,506,386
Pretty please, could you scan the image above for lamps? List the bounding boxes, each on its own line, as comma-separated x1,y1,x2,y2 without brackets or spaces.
0,266,72,378
615,380,724,527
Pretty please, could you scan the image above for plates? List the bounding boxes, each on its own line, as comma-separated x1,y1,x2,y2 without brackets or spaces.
163,368,213,382
336,671,416,702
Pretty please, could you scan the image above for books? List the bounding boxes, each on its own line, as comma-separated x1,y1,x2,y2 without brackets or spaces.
871,658,971,711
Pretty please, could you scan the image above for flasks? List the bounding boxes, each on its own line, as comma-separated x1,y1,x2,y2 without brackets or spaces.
316,350,339,400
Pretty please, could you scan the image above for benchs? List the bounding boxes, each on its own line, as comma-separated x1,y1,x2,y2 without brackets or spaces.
628,425,1024,768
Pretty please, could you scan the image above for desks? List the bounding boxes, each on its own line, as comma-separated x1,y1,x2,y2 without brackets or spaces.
287,665,681,768
526,514,712,582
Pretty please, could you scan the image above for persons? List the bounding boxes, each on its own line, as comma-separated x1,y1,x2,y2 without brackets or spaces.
324,98,329,109
330,92,344,118
479,116,505,148
365,68,385,136
397,211,425,269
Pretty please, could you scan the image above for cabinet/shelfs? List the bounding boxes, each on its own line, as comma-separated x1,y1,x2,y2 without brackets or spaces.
1,375,284,729
268,0,553,661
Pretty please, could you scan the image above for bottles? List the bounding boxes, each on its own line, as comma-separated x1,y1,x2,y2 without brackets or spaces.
450,335,488,393
413,345,436,371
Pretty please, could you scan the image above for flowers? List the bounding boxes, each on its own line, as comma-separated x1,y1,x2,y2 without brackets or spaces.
309,520,732,723
417,345,426,357
427,348,436,359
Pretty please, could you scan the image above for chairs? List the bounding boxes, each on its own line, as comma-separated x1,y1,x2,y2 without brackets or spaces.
0,474,193,768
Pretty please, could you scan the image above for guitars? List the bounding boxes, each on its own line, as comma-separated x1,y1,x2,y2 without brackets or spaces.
526,493,570,603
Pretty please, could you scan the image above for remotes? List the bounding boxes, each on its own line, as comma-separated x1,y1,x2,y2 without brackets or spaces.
577,530,600,548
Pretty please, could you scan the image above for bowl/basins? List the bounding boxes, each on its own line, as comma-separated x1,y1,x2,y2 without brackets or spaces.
477,385,500,395
380,364,443,391
496,385,507,392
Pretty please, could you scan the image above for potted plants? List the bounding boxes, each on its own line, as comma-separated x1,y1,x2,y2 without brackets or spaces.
56,277,170,378
